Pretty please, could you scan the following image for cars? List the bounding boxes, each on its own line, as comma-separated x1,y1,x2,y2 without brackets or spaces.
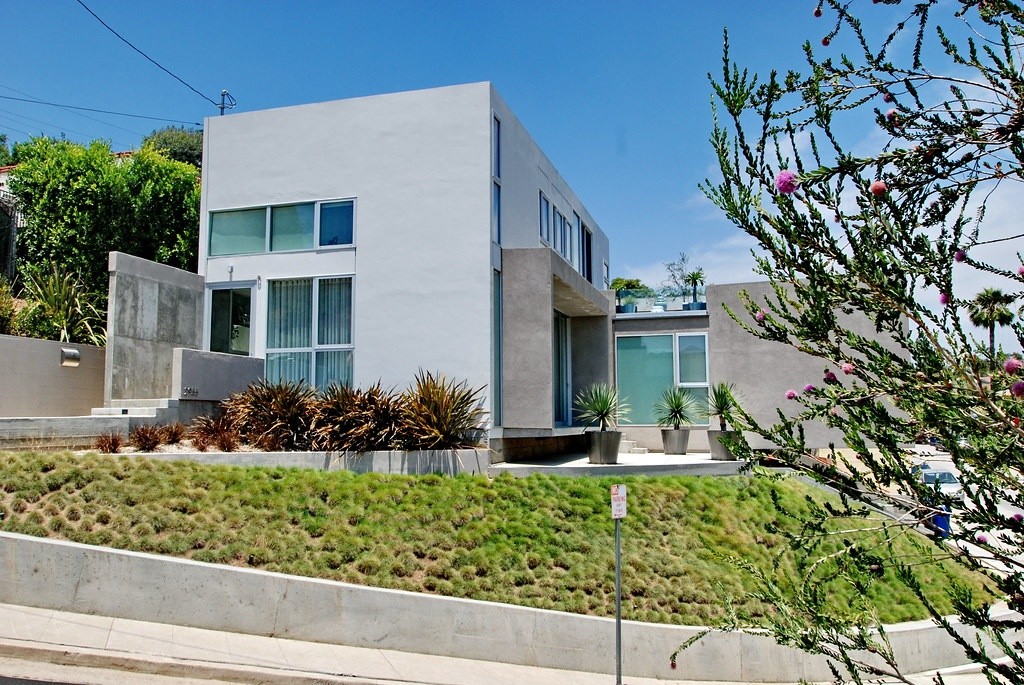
915,468,964,507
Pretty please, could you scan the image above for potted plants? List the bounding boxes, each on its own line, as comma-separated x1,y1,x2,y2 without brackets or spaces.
609,277,656,313
644,286,673,311
683,266,706,310
621,294,640,313
570,381,632,464
651,384,701,455
658,251,690,311
693,380,748,461
